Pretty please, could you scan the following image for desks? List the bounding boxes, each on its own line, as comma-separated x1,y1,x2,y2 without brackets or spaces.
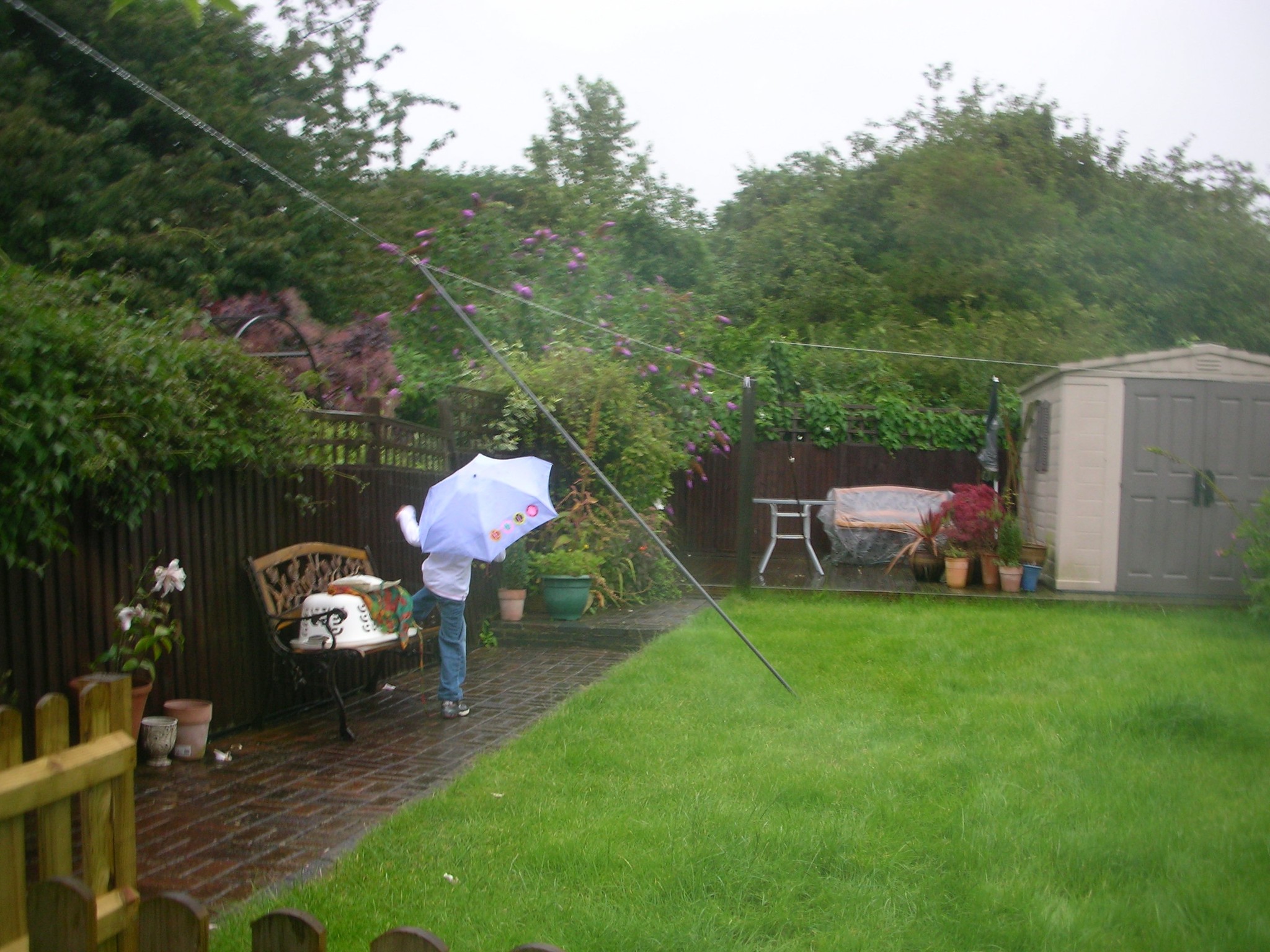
753,499,836,574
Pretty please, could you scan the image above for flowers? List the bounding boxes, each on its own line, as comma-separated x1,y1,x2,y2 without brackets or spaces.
935,481,1002,550
83,543,187,684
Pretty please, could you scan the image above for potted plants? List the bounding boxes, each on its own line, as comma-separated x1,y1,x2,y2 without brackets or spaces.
998,515,1024,591
980,509,999,585
884,506,947,581
499,540,530,621
529,550,605,622
941,543,971,589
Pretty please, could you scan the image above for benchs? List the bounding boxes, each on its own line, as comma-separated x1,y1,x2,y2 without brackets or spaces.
244,540,444,742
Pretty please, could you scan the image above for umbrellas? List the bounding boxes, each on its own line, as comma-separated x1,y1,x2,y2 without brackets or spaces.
417,451,559,565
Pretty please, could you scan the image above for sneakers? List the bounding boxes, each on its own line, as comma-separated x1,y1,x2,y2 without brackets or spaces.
411,617,425,630
440,700,469,717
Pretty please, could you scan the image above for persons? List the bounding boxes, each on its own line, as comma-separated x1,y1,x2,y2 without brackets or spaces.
394,506,506,720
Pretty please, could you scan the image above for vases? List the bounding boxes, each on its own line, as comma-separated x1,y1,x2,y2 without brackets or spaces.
1021,562,1043,592
1020,539,1048,571
166,699,213,760
65,674,153,745
143,715,177,768
